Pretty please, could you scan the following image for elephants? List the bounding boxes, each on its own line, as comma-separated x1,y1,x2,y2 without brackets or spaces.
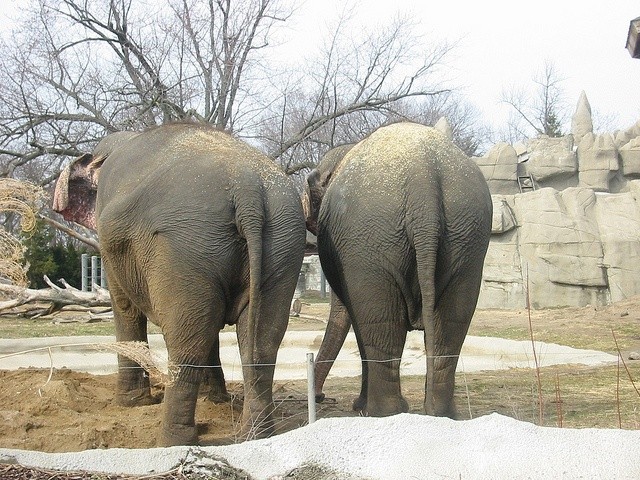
51,122,306,447
301,120,493,418
